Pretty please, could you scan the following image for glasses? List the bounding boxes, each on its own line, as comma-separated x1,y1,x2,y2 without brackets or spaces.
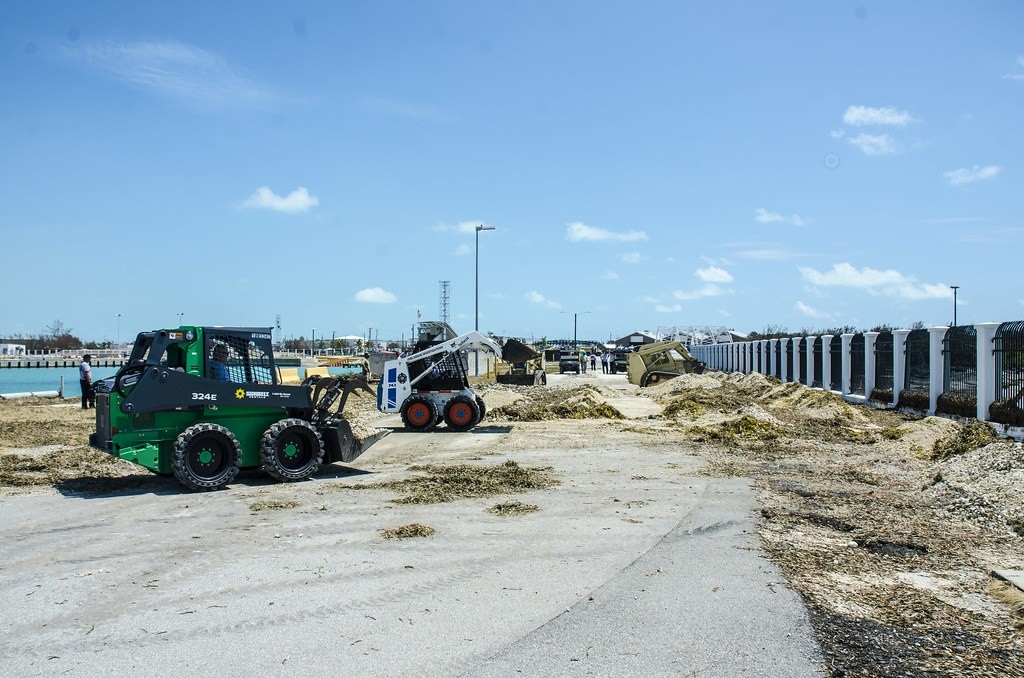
219,351,229,356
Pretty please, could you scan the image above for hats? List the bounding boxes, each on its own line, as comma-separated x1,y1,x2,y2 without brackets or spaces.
83,354,92,358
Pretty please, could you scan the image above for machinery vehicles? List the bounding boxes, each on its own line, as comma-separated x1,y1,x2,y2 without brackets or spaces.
627,341,707,388
89,325,391,491
496,344,548,386
377,331,543,434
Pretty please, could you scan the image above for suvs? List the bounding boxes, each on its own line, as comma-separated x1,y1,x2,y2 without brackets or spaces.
610,352,628,374
559,350,580,374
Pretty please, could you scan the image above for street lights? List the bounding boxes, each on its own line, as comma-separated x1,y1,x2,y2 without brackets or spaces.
115,313,121,350
475,223,496,332
559,311,591,351
177,312,184,326
950,286,960,326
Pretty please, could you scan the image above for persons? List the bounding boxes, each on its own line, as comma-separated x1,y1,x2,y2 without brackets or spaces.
581,352,588,373
600,350,608,374
609,351,615,373
210,344,232,382
396,349,408,359
361,352,370,391
79,354,96,409
590,352,596,370
